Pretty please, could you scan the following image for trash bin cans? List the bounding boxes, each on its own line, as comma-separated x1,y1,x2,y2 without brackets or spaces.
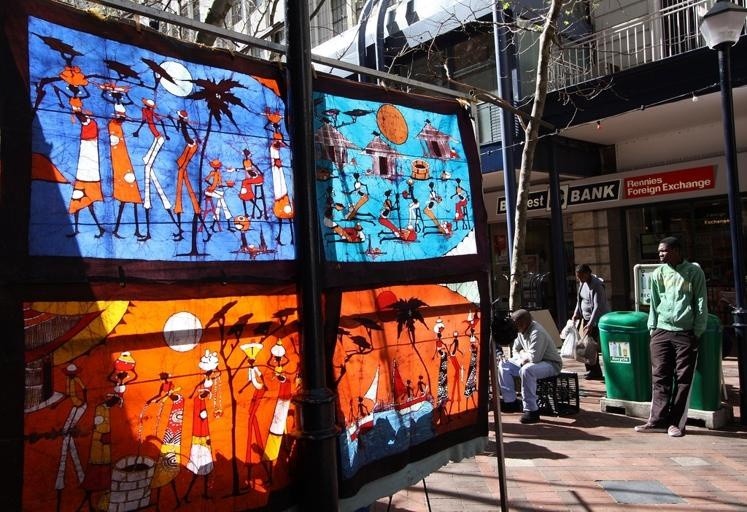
597,311,651,401
669,312,725,411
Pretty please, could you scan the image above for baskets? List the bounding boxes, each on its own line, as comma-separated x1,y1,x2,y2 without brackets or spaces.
536,373,580,418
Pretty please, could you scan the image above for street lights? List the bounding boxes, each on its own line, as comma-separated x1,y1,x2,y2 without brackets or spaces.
697,1,746,428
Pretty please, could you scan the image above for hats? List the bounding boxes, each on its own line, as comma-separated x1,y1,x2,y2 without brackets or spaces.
142,98,157,108
177,110,189,120
209,160,222,168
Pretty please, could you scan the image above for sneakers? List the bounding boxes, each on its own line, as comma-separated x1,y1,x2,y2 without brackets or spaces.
634,422,683,437
584,370,603,380
520,410,540,424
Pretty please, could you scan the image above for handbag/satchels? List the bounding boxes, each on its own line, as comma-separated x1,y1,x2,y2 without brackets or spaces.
560,319,599,366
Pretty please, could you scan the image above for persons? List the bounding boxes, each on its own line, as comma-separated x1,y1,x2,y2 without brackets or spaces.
566,264,612,381
495,308,563,424
635,236,709,438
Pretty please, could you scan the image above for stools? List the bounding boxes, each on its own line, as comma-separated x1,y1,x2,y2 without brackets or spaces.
514,372,580,417
